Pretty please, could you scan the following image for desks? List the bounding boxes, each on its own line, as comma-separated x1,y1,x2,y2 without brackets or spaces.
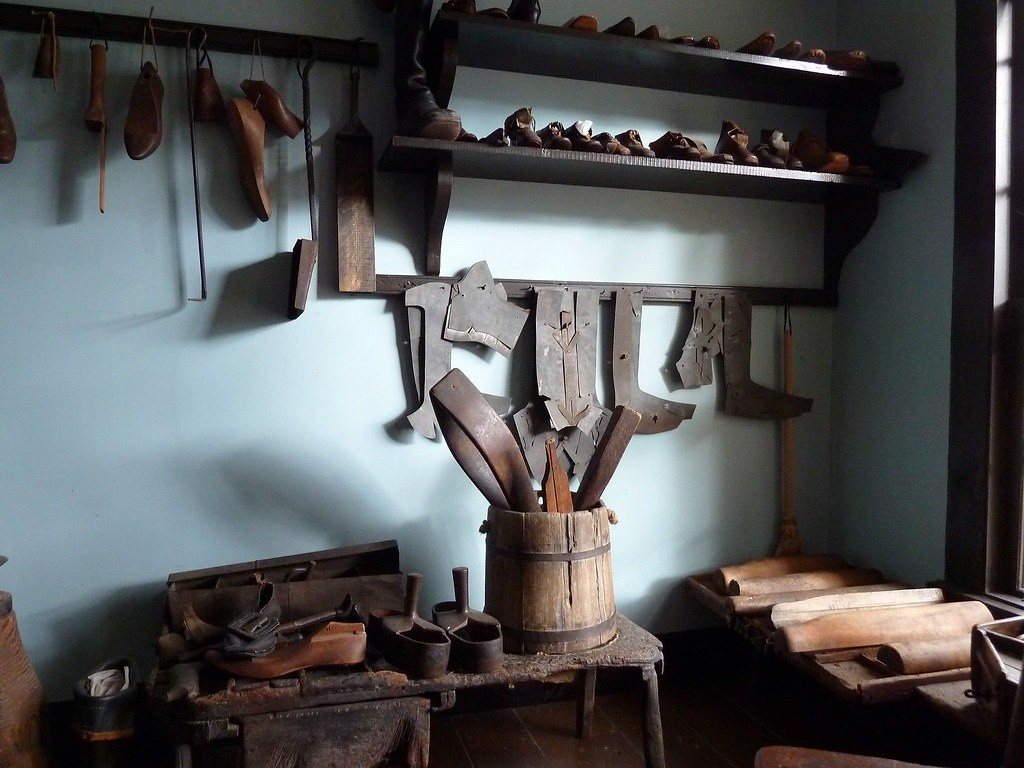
155,610,669,768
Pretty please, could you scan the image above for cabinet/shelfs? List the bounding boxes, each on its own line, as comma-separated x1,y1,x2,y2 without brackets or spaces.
374,12,931,308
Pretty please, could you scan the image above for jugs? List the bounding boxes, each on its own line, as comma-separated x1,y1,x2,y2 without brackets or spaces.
70,655,138,768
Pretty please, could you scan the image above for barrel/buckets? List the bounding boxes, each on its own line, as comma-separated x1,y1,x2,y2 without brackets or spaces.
478,490,617,654
